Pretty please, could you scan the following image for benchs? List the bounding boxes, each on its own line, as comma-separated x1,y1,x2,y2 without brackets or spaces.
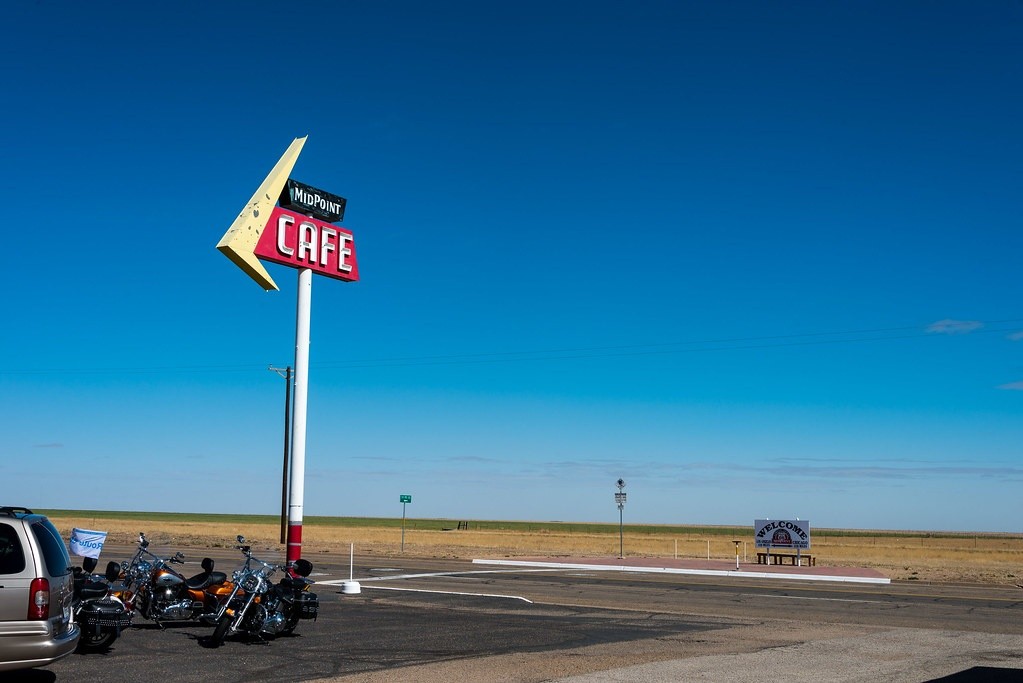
757,552,816,567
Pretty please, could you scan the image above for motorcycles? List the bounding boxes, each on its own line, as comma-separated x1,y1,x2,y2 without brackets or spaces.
212,535,320,648
113,532,244,628
67,555,132,652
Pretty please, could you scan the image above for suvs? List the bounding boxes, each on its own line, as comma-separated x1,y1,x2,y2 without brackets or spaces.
0,505,80,674
